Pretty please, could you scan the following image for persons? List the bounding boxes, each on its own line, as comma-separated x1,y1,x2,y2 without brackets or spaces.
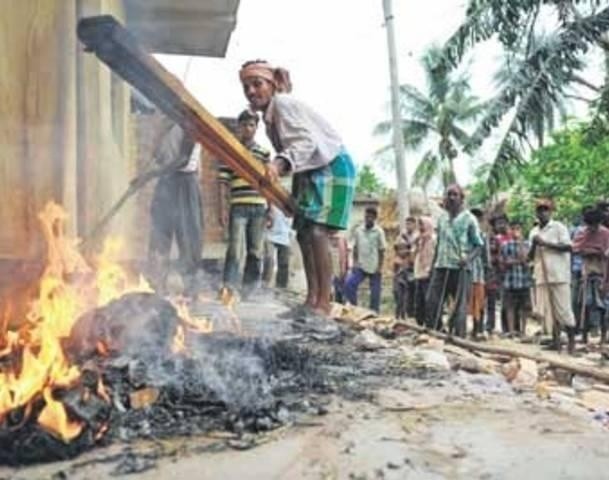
240,57,356,330
218,110,274,300
327,227,348,303
344,207,386,316
129,108,206,299
391,183,608,357
261,171,291,288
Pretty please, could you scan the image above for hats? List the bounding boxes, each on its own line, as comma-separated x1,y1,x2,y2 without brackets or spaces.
535,199,552,210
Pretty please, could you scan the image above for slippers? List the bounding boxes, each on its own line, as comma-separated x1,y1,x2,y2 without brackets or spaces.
275,304,309,320
291,310,325,328
